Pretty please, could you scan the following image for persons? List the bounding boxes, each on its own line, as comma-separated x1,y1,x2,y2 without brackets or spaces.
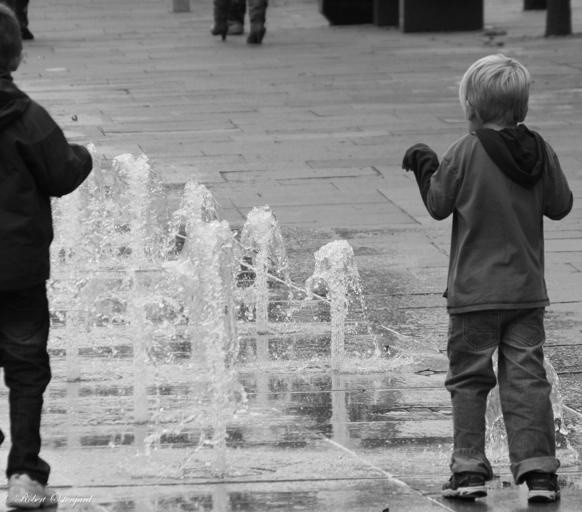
210,0,267,43
0,3,93,508
400,53,572,503
0,0,34,39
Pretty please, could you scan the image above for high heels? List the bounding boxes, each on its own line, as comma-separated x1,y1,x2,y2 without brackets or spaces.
211,22,265,44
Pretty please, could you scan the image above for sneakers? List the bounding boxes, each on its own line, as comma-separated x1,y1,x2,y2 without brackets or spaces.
6,475,55,507
527,469,560,501
442,470,488,497
18,27,34,40
